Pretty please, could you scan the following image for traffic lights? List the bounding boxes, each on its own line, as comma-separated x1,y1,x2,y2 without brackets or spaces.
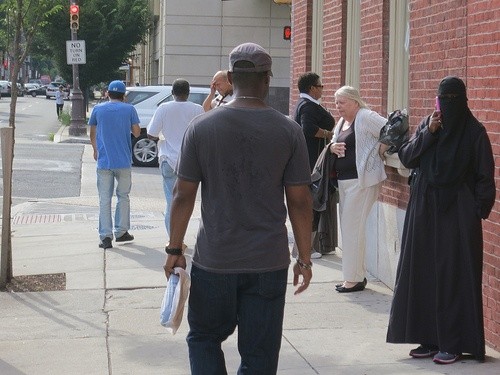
70,3,80,30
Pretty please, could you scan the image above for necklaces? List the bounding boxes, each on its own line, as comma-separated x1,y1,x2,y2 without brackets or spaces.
232,95,262,102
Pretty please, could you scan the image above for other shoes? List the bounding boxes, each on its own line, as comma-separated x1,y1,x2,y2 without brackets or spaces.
325,251,336,254
116,231,134,242
310,252,322,259
99,237,112,248
335,277,368,292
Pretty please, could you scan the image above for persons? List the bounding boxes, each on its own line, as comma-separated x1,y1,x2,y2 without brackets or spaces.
88,79,141,248
162,43,314,375
147,79,203,248
329,85,400,292
55,85,64,118
385,77,495,364
202,70,236,110
294,72,335,259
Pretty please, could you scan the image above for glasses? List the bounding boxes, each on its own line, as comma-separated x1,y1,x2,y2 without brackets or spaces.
311,84,323,89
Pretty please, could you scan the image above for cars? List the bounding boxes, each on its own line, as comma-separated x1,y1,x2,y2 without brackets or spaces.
0,74,72,101
92,86,108,99
123,85,219,167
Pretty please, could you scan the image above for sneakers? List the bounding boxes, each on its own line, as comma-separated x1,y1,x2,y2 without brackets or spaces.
409,344,438,356
434,351,463,363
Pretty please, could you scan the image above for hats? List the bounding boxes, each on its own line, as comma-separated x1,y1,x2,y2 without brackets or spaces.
228,42,273,77
108,80,126,94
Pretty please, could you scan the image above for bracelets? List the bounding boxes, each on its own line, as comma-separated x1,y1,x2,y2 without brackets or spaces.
165,246,182,256
297,260,312,269
323,130,326,138
